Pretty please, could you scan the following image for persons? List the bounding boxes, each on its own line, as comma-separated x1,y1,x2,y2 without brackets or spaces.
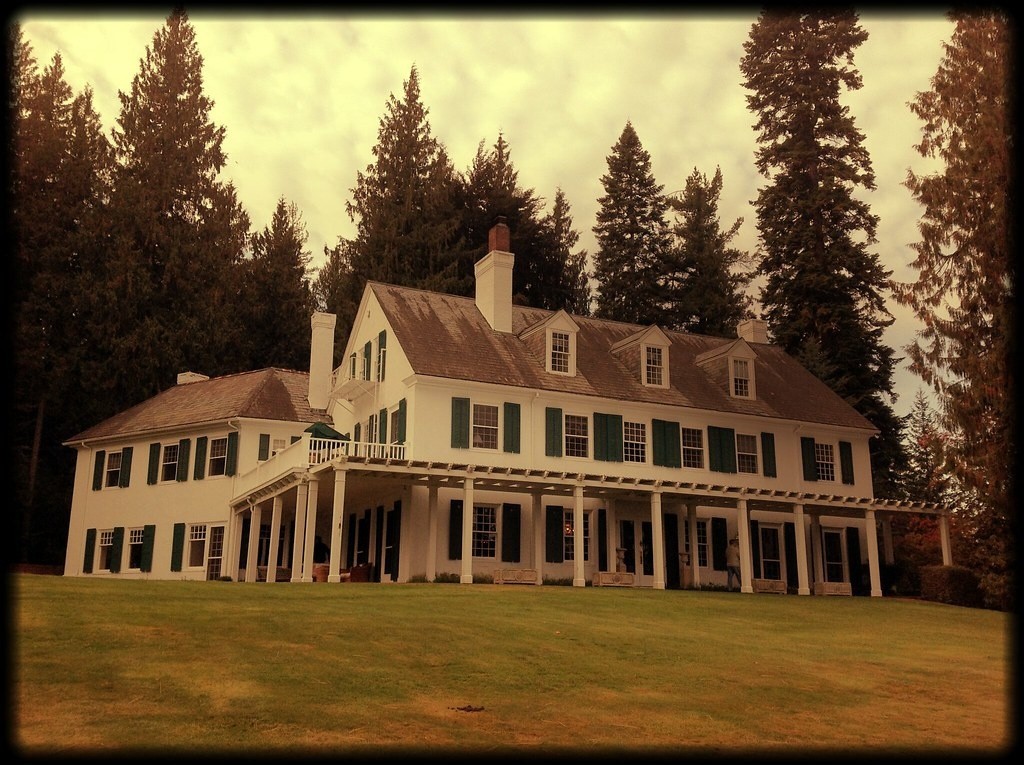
313,535,331,564
726,539,741,591
